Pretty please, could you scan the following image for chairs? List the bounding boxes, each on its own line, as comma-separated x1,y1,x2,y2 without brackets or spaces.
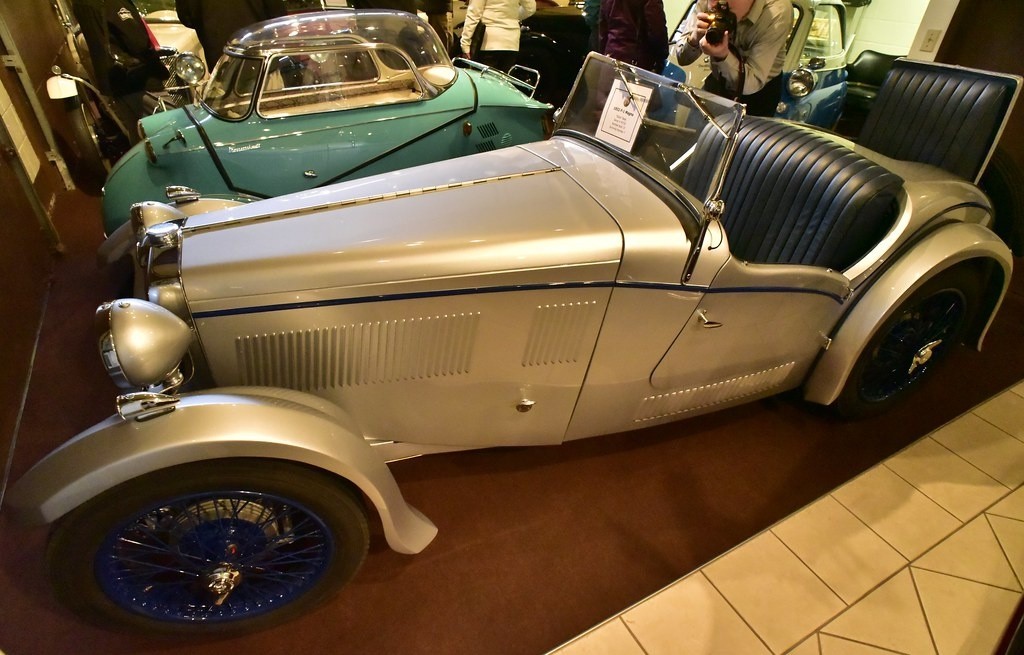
870,55,1024,185
821,50,906,120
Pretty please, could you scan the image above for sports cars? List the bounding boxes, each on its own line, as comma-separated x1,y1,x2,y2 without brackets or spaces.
0,48,1024,641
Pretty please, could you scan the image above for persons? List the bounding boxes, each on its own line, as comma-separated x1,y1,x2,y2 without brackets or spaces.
71,0,171,144
176,0,668,146
675,0,794,138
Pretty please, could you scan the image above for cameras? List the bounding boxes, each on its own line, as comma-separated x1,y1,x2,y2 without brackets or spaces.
704,6,738,44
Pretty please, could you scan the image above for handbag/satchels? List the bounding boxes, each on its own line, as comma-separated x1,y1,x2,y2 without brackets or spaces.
108,51,170,96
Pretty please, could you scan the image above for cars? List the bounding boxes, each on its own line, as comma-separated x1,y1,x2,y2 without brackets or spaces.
635,0,856,149
101,8,557,240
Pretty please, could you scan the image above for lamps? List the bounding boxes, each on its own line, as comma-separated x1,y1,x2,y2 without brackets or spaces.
47,63,130,138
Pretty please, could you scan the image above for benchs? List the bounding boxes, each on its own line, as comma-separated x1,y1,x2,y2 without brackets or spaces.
688,110,900,272
